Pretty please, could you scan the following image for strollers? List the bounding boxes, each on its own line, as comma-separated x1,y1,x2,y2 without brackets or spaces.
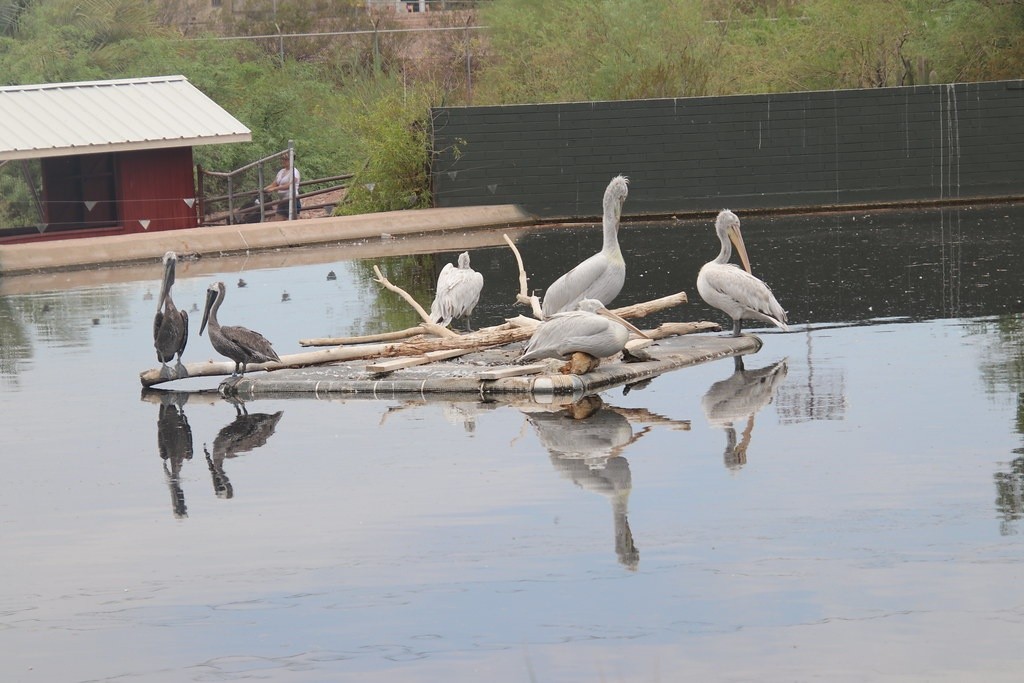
226,187,272,225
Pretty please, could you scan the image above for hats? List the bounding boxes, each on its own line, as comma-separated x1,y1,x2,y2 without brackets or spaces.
278,152,295,160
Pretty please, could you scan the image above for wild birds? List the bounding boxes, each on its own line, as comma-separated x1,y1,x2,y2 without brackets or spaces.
697,208,791,338
198,281,283,377
429,251,484,332
539,171,629,317
153,251,190,380
514,298,652,362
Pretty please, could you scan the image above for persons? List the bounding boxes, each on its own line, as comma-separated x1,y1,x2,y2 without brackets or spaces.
264,152,301,222
